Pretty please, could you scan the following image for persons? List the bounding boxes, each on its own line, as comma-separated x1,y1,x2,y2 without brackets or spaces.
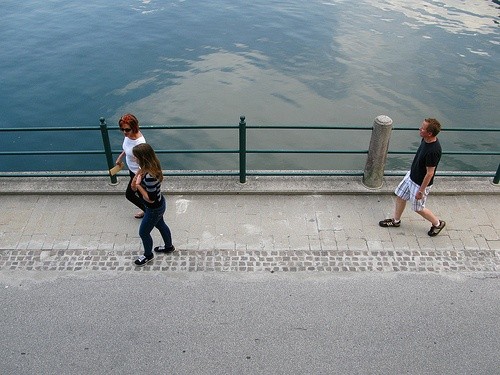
379,119,445,237
131,143,175,265
115,115,149,218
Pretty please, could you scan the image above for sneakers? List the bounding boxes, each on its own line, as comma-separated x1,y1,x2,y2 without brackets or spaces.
134,253,155,266
427,220,446,237
154,244,175,253
379,218,401,227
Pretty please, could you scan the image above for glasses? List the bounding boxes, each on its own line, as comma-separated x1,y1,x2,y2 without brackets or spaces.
120,127,132,132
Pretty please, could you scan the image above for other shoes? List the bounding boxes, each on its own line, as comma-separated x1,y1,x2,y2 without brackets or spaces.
134,210,144,219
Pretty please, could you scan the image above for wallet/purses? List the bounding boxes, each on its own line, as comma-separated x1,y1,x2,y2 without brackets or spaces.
109,164,122,176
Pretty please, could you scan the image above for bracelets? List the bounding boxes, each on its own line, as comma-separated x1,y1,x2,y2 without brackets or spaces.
419,189,424,194
